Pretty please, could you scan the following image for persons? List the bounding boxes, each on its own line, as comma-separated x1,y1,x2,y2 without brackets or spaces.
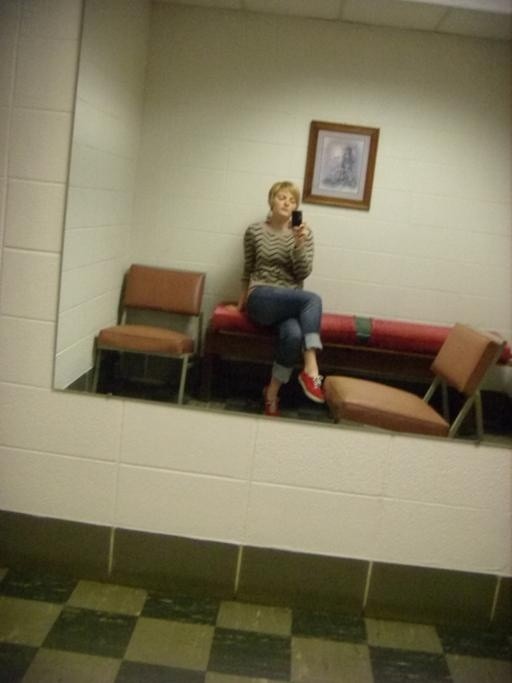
223,181,324,417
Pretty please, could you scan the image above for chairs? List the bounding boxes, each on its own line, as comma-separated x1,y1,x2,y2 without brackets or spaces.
91,263,205,406
323,323,507,444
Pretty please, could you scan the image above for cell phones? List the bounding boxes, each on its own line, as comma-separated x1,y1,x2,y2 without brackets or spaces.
292,210,302,226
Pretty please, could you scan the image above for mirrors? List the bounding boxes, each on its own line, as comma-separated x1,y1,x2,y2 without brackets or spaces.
52,0,512,449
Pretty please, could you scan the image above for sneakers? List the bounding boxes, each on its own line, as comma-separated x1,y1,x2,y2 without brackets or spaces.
262,384,283,418
295,369,326,405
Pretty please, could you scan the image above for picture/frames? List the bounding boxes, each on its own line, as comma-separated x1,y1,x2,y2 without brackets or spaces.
302,119,379,211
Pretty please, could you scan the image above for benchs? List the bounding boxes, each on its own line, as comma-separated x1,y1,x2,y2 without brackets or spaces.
200,301,511,403
